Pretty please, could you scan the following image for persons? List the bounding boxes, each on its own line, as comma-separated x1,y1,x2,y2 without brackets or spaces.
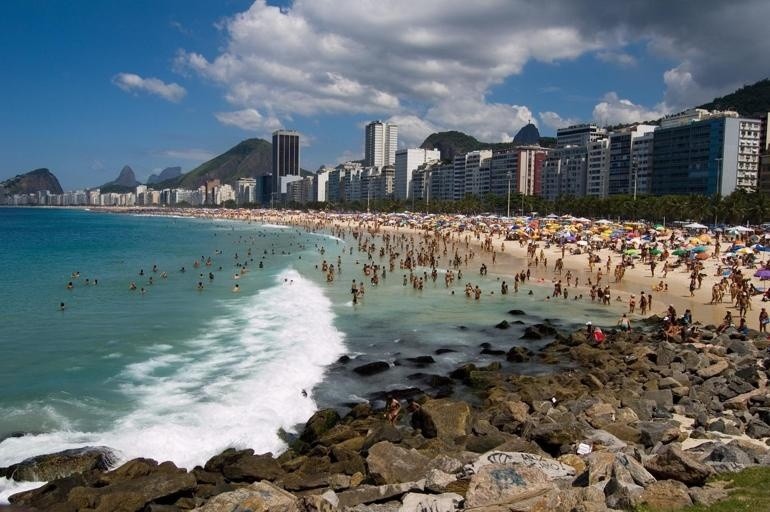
406,395,420,413
384,391,402,428
